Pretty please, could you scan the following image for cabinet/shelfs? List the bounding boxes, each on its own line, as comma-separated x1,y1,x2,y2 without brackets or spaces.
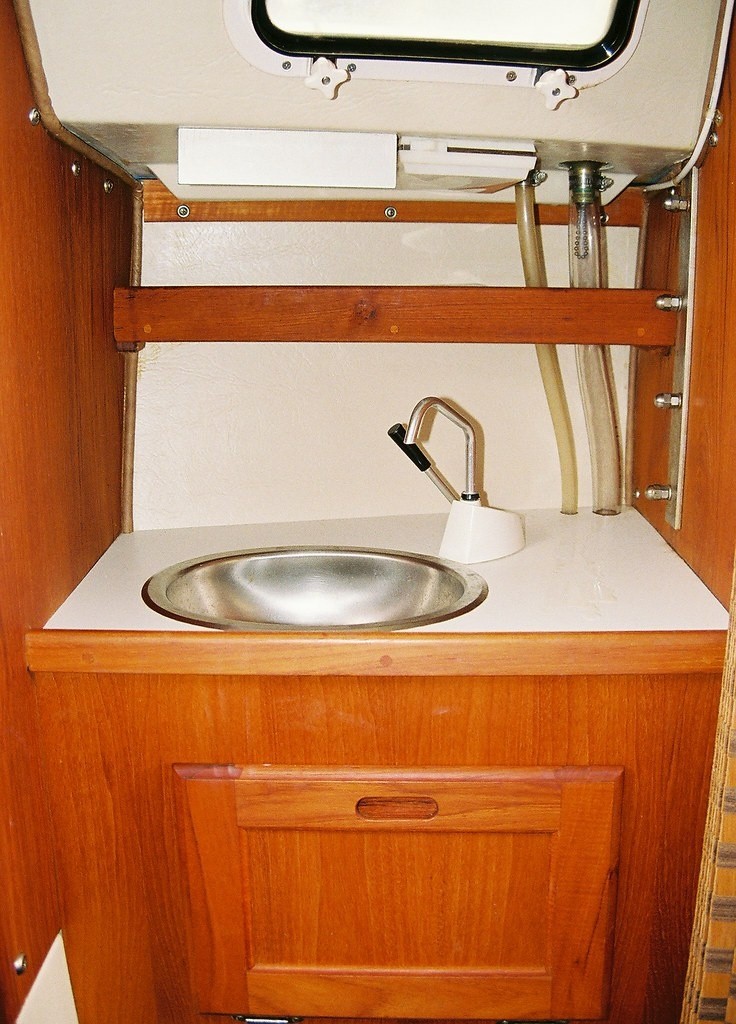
28,662,725,1024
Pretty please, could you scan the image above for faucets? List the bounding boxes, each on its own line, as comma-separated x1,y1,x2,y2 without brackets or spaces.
386,395,528,566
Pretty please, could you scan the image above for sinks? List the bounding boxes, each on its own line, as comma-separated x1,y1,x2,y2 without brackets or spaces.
140,544,493,631
